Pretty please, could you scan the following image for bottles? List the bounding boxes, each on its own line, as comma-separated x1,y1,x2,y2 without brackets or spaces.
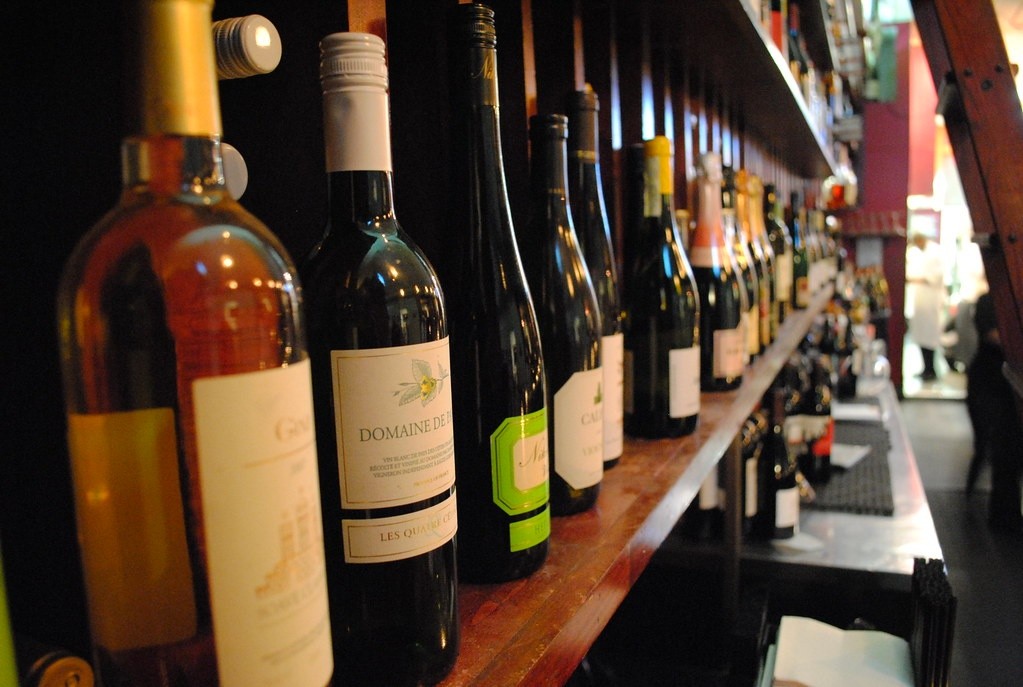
0,0,889,686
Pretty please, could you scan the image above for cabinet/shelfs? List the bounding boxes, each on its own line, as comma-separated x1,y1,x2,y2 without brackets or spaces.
0,0,958,687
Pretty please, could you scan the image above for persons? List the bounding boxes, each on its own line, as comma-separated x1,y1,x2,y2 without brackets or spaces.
913,267,1009,502
